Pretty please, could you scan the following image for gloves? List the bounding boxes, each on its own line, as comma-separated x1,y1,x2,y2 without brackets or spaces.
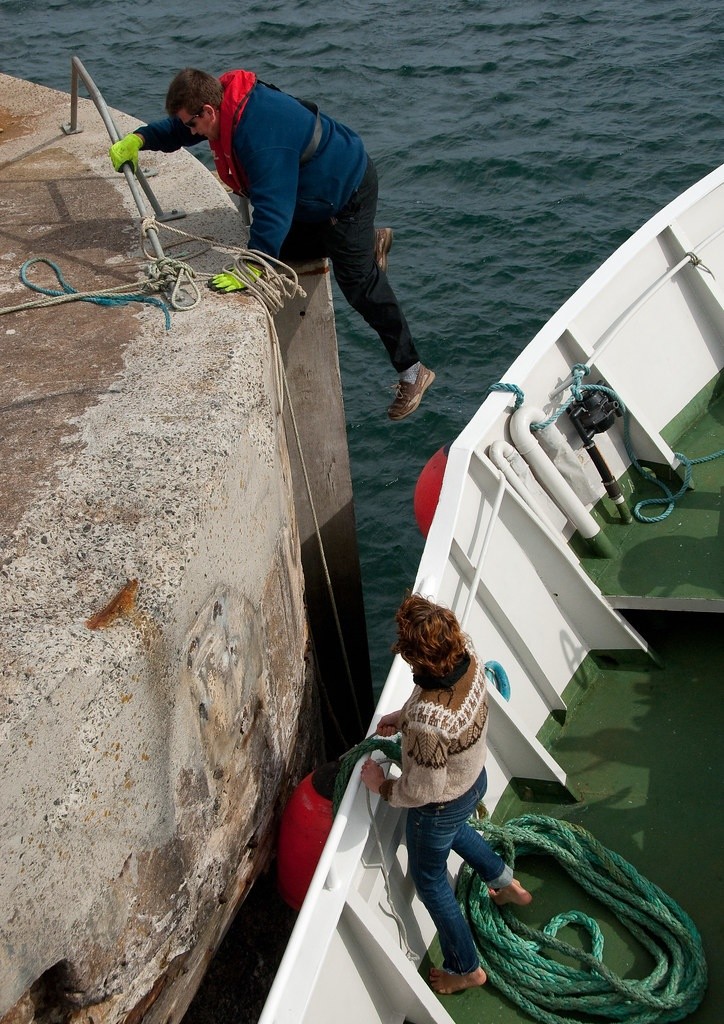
207,261,262,294
109,133,144,175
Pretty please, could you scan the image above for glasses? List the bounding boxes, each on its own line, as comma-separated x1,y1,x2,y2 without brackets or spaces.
184,108,203,128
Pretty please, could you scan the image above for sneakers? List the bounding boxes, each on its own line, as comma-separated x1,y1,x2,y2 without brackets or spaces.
388,364,436,420
375,228,393,275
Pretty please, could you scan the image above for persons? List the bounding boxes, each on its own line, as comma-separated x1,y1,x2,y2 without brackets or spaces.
373,592,533,996
108,66,436,421
